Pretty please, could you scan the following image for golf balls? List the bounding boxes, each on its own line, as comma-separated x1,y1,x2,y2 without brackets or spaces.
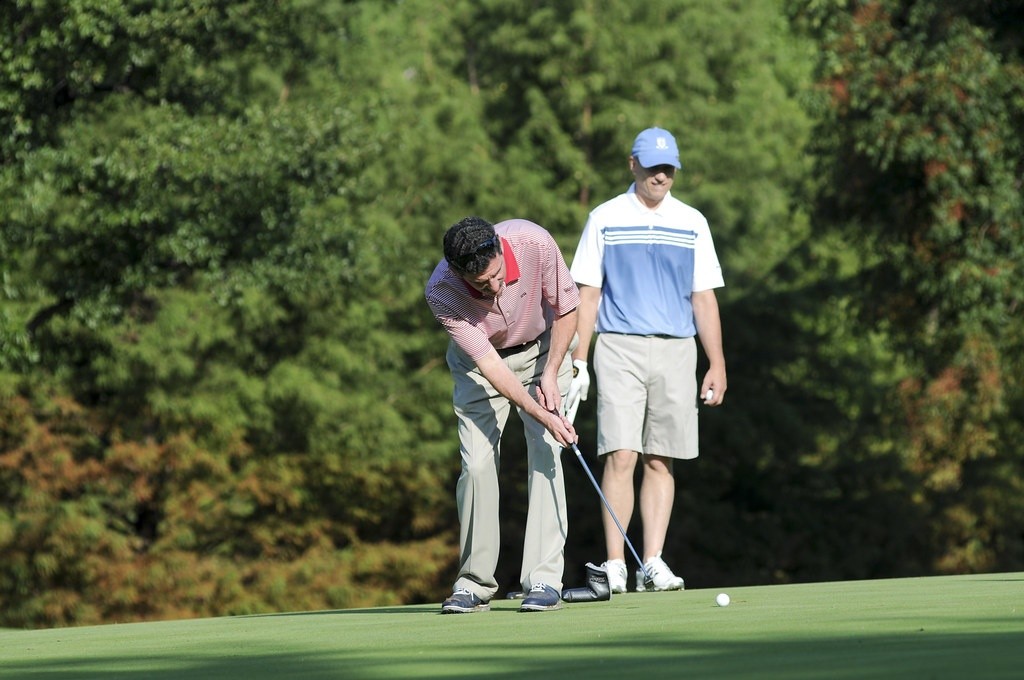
716,593,730,607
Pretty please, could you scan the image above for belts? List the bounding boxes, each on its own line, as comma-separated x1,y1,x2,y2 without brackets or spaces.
609,330,680,340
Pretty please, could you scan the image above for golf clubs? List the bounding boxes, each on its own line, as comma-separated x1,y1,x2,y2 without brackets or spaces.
534,379,655,591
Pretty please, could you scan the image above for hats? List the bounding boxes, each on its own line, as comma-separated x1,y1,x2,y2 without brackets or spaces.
631,127,681,170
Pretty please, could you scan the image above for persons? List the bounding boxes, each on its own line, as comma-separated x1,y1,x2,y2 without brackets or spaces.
565,127,728,590
426,216,582,612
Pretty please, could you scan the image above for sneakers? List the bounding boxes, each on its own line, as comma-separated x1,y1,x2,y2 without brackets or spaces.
521,584,560,611
600,559,628,593
636,558,684,593
443,591,490,614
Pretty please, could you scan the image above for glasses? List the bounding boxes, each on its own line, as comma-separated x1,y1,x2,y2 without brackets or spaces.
451,236,496,269
